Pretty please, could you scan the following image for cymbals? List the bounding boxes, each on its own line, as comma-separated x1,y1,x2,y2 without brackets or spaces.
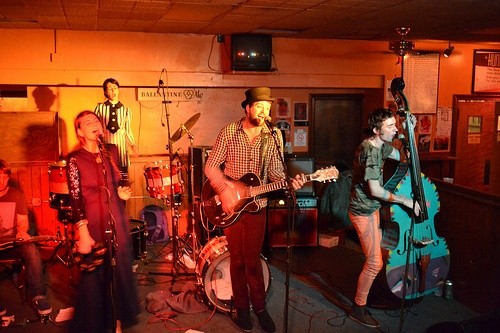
169,113,203,140
72,241,107,271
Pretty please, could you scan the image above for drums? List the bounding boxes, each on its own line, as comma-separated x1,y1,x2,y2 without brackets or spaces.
111,219,148,261
196,235,269,314
143,159,184,200
46,161,72,210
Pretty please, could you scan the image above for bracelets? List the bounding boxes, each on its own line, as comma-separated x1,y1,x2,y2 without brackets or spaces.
75,219,88,229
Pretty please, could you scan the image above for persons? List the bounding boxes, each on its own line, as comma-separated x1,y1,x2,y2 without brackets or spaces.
93,78,137,166
66,110,141,333
347,108,421,328
203,87,307,333
0,160,52,322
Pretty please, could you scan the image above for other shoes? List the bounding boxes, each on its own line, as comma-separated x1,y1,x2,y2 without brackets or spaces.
256,310,275,333
238,303,252,330
348,302,380,328
367,296,387,307
34,299,52,314
0,307,6,316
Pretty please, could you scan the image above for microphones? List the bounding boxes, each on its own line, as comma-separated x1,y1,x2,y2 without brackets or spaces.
157,70,165,93
398,134,411,153
172,149,179,161
264,116,276,134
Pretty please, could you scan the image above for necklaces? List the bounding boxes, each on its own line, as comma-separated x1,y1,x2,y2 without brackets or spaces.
82,145,102,163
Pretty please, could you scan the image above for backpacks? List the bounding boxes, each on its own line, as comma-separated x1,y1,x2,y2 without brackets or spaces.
142,204,169,245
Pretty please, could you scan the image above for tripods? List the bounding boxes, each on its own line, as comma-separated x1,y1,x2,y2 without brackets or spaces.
43,218,77,289
147,78,203,293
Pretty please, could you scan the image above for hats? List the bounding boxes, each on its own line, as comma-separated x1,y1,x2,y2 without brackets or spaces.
241,87,275,109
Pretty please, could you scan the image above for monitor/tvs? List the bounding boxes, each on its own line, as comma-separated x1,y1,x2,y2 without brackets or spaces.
230,33,272,71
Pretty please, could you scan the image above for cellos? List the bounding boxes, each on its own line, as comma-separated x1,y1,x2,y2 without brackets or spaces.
379,76,451,300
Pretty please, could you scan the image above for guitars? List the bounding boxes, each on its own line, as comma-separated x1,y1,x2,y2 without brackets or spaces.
201,165,339,228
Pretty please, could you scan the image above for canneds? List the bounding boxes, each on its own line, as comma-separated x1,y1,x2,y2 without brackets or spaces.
444,280,453,299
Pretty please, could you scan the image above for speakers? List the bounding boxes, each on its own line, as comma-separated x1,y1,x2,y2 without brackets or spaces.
187,144,214,202
267,207,319,249
269,157,314,196
